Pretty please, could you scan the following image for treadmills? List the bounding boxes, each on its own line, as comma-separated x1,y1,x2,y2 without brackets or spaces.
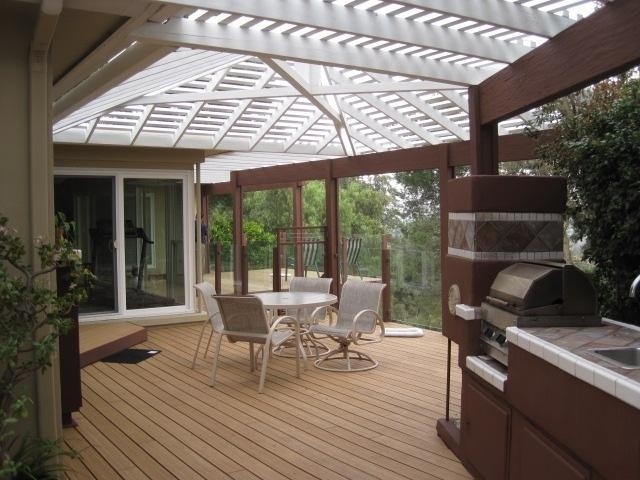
89,220,175,309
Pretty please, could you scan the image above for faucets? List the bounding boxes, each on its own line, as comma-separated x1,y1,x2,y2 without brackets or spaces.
629,274,640,299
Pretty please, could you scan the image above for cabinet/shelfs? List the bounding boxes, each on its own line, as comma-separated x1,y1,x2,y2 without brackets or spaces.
458,376,512,480
509,342,640,479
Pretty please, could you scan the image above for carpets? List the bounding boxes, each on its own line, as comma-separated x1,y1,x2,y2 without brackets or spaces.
100,348,161,365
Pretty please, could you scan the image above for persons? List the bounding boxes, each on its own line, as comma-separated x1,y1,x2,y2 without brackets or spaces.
195,211,208,297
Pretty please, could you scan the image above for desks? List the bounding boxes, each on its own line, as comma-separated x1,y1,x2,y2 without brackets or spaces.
245,292,338,370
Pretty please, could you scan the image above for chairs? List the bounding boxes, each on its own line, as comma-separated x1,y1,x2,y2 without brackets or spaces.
271,277,334,358
308,279,387,372
284,236,363,281
190,281,271,372
207,294,302,394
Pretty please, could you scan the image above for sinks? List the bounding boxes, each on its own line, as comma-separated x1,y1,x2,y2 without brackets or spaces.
590,347,640,368
